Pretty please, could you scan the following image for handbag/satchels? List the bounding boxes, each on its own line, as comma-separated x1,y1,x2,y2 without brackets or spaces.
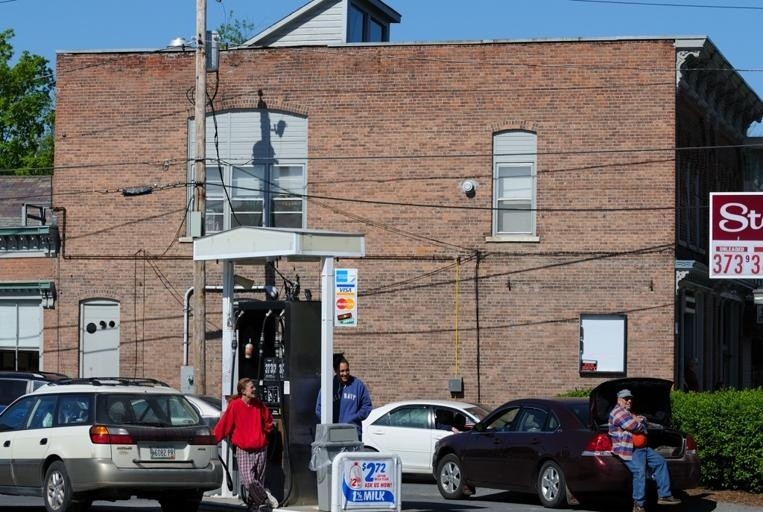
631,432,648,449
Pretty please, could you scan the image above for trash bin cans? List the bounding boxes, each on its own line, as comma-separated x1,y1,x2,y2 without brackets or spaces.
311,423,364,512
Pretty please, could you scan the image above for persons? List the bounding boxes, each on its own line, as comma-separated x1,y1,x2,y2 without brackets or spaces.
435,410,459,434
212,377,279,511
608,389,682,511
314,356,372,443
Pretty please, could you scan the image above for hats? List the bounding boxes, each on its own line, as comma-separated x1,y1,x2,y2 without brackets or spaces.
616,389,633,399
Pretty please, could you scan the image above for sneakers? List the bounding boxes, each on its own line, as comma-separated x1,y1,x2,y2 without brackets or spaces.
657,496,682,505
263,487,279,508
632,502,647,512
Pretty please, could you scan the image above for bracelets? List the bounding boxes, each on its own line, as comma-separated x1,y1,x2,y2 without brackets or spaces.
454,428,456,431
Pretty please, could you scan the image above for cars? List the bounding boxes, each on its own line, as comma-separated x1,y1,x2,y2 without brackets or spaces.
0,370,222,512
